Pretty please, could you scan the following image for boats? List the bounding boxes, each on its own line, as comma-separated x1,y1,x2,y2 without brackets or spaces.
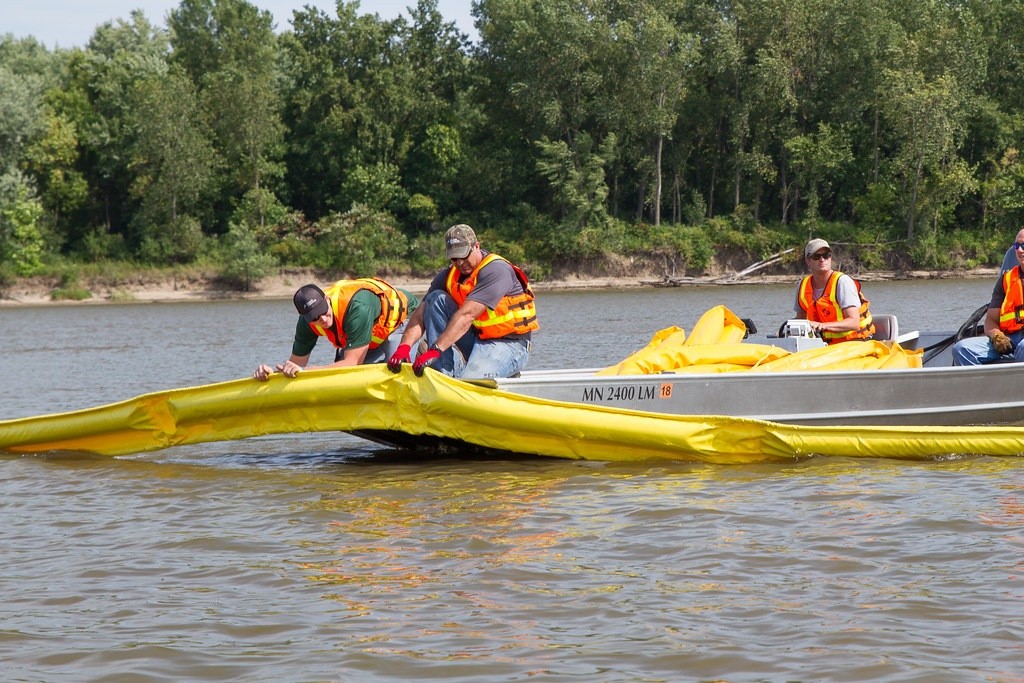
342,355,1024,459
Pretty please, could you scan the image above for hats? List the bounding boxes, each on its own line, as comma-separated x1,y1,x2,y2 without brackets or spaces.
293,284,328,324
805,239,832,259
443,224,477,259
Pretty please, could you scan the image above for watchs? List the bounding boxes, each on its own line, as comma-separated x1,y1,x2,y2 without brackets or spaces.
430,344,443,355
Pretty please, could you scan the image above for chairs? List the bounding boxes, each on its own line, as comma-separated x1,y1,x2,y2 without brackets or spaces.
871,313,919,352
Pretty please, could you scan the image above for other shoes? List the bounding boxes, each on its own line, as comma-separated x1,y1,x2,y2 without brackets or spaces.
508,371,521,378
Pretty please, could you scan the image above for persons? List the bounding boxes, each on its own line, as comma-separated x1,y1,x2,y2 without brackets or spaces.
252,276,426,382
792,237,876,345
388,225,539,382
954,227,1024,365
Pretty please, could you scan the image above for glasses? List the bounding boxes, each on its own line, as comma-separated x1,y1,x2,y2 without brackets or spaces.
807,251,832,260
1012,242,1024,249
450,242,475,262
311,311,327,321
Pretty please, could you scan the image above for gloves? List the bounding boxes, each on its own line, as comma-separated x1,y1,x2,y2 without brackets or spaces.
388,344,411,374
412,349,441,377
989,329,1016,354
